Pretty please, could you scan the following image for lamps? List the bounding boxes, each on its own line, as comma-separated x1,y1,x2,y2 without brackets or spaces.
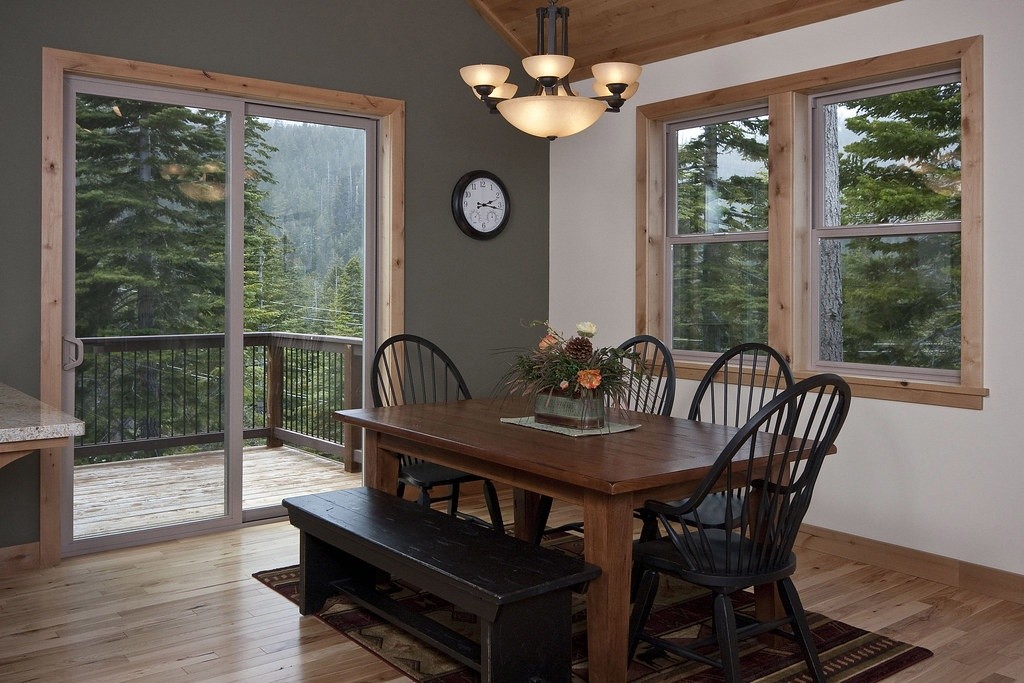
460,0,643,142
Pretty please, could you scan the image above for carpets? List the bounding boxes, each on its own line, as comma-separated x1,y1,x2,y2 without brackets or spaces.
251,523,934,683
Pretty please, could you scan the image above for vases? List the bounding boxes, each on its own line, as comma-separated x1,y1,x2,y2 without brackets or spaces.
534,389,605,429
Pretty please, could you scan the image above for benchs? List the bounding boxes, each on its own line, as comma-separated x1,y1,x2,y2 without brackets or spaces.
282,486,603,683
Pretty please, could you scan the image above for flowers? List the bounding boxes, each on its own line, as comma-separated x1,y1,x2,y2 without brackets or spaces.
492,314,663,437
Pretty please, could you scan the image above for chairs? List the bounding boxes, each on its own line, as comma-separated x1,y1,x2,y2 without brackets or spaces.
628,371,853,683
657,342,797,532
369,333,506,535
601,335,676,417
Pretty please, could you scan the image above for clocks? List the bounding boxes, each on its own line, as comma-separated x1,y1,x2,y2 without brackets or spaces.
451,170,511,241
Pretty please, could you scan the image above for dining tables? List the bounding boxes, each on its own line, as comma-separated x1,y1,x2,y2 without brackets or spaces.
333,398,838,683
